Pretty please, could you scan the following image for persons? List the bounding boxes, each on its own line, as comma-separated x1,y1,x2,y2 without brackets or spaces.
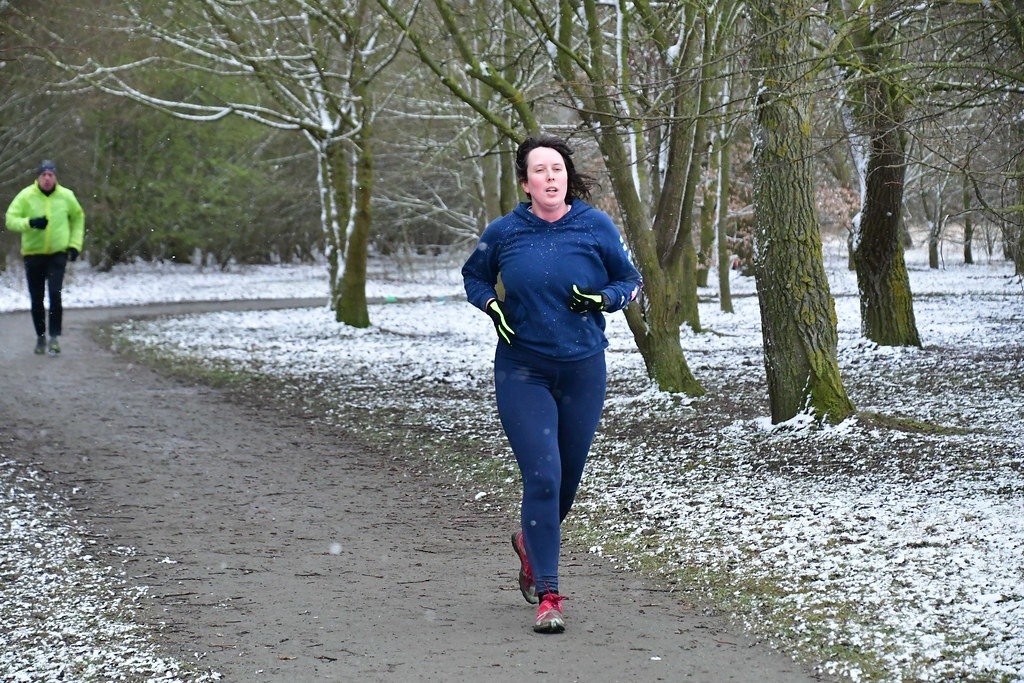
5,160,87,357
459,137,644,634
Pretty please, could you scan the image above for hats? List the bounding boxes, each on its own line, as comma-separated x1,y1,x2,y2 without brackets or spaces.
38,159,57,176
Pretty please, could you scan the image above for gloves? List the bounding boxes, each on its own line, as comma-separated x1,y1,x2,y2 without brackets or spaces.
487,300,517,348
67,248,77,261
30,216,48,229
568,284,604,314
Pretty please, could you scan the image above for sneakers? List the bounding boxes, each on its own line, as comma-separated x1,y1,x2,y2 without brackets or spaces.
35,336,60,356
510,527,568,635
